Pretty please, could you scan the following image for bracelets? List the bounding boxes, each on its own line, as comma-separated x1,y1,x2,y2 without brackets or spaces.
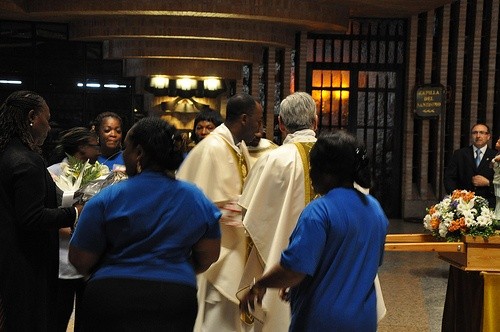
488,180,490,186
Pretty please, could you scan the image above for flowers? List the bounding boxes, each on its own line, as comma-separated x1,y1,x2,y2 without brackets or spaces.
60,153,127,192
421,188,498,241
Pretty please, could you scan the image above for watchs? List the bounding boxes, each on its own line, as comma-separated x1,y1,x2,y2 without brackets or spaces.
249,277,266,295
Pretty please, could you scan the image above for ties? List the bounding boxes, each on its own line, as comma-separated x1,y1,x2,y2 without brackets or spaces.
476,149,482,168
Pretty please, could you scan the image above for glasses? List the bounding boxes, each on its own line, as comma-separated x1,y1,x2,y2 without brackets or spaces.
471,131,489,135
86,143,100,151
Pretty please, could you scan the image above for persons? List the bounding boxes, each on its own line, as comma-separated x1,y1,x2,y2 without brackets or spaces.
45,126,111,332
90,110,125,172
175,97,284,332
443,124,496,212
239,131,391,332
231,93,387,332
67,118,223,332
0,92,88,332
